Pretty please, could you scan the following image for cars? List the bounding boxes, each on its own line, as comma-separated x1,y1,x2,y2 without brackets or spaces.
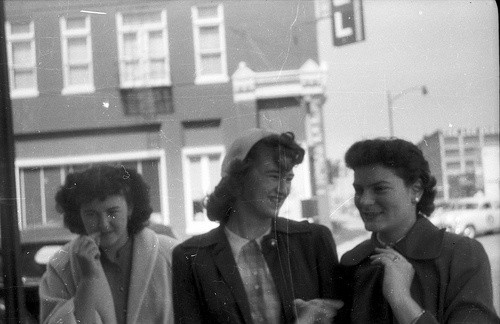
424,200,458,230
2,220,177,323
439,196,500,241
330,201,374,239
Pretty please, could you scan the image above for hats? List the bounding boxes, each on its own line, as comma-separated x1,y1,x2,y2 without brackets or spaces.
219,127,282,177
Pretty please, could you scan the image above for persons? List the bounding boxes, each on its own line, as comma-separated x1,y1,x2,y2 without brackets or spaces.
39,165,185,324
294,136,470,323
171,129,343,324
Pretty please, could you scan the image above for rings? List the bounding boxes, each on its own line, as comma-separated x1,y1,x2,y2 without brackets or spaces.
392,254,401,263
